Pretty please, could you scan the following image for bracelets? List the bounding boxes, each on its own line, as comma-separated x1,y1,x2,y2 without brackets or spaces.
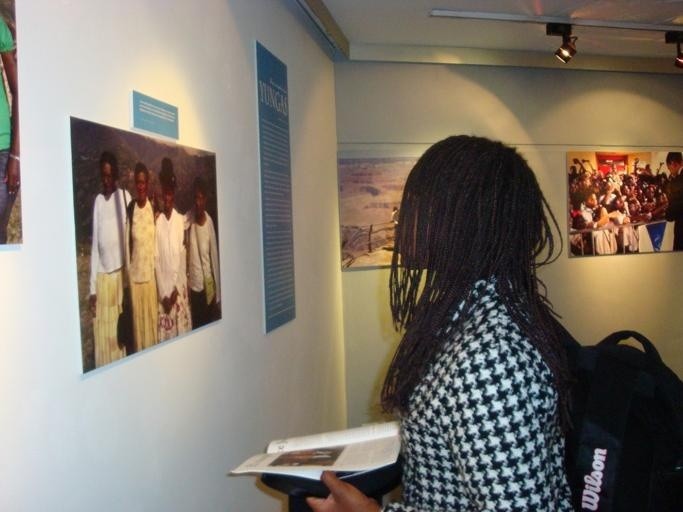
9,154,20,162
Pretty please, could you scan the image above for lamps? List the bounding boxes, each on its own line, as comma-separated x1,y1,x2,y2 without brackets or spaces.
544,19,579,65
662,28,683,68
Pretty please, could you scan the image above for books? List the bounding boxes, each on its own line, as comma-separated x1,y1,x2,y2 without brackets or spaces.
228,420,402,483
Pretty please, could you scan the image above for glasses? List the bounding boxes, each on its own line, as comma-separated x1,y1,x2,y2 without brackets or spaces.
390,206,403,227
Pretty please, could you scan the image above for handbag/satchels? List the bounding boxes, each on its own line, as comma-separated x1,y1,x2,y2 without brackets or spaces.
204,274,215,305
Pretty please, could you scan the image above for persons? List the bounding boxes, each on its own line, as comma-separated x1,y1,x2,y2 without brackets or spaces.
566,151,683,255
307,134,574,512
87,151,220,369
0,0,23,245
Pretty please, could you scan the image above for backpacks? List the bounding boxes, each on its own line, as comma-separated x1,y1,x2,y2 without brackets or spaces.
512,279,682,508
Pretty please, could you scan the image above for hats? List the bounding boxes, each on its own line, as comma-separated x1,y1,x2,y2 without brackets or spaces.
159,157,178,187
